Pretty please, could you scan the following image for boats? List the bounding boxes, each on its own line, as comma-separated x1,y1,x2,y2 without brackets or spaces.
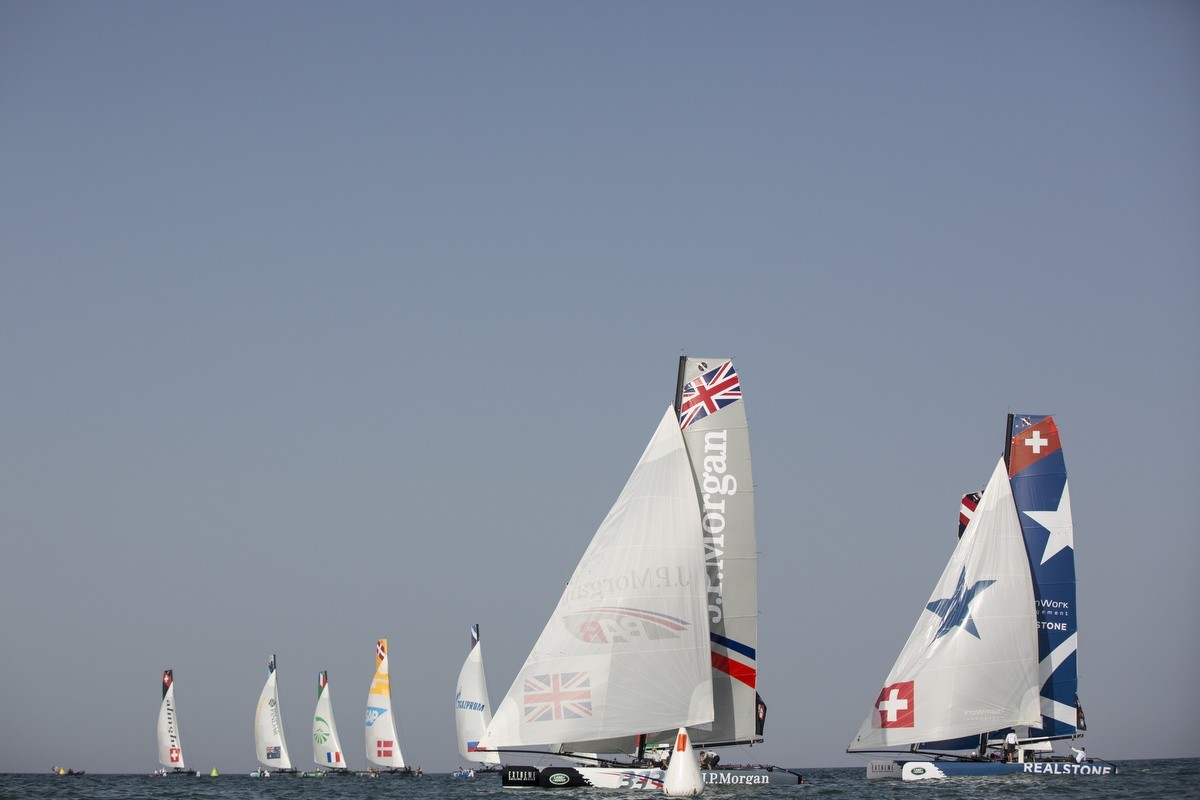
51,764,88,776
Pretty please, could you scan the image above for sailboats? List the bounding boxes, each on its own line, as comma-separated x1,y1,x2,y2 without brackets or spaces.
465,351,810,789
450,621,512,781
350,637,422,779
152,667,200,777
847,411,1123,780
247,653,306,778
302,669,359,781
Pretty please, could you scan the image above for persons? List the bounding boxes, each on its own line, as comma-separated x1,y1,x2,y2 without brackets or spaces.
698,750,720,770
1004,729,1021,763
1068,745,1086,764
